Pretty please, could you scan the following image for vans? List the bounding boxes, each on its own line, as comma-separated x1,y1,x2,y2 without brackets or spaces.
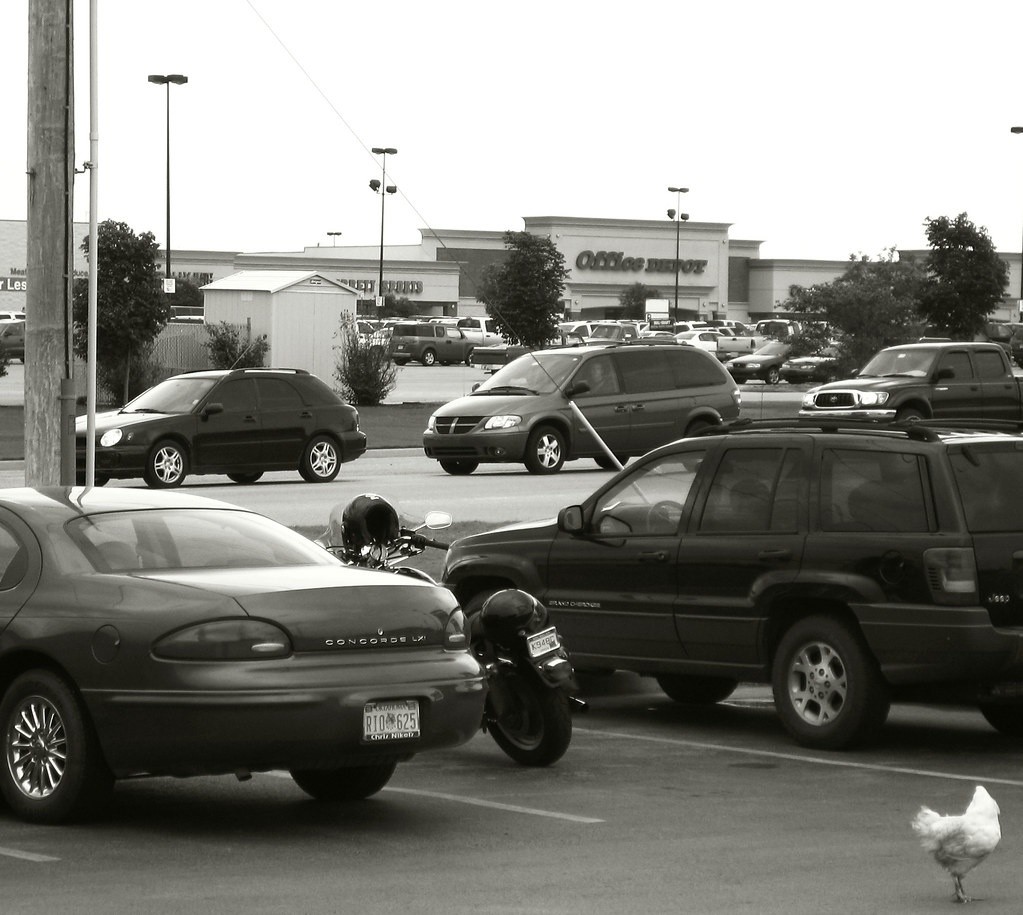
422,340,742,475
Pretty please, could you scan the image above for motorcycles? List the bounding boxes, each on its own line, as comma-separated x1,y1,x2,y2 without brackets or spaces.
311,500,588,768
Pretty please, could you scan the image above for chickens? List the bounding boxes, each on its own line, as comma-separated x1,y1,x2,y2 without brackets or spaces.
910,786,1002,903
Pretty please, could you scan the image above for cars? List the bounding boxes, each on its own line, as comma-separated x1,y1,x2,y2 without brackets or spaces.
780,343,840,384
0,484,489,823
75,344,368,490
385,322,483,366
357,315,833,361
722,340,794,385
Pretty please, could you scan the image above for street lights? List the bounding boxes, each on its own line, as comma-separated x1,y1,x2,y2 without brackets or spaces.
149,74,188,323
327,231,343,245
369,147,397,320
667,186,689,321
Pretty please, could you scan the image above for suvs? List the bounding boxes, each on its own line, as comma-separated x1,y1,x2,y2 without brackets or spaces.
442,415,1023,747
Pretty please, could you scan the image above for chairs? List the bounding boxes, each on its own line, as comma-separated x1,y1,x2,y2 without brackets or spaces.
846,476,905,536
98,539,139,574
729,479,778,535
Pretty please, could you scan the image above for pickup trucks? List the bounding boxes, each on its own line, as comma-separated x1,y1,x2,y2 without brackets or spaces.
470,331,586,376
797,343,1023,422
